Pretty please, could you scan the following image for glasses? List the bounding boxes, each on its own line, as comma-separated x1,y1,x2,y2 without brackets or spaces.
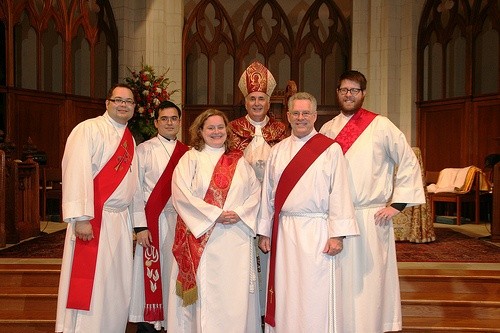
157,117,180,122
339,88,363,95
109,97,135,106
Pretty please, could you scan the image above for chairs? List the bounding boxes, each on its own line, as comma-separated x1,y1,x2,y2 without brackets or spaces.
427,166,491,225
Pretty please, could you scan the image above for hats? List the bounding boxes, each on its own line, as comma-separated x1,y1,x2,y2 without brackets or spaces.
238,61,277,98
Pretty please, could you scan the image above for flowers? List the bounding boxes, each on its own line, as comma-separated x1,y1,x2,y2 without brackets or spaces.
119,55,184,137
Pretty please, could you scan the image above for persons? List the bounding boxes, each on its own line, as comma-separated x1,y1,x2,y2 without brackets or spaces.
318,70,426,333
256,92,360,333
55,84,139,333
167,109,262,333
224,61,292,333
129,101,193,333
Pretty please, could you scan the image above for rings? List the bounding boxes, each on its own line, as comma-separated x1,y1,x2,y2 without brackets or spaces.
139,242,143,245
383,215,387,220
225,218,231,222
